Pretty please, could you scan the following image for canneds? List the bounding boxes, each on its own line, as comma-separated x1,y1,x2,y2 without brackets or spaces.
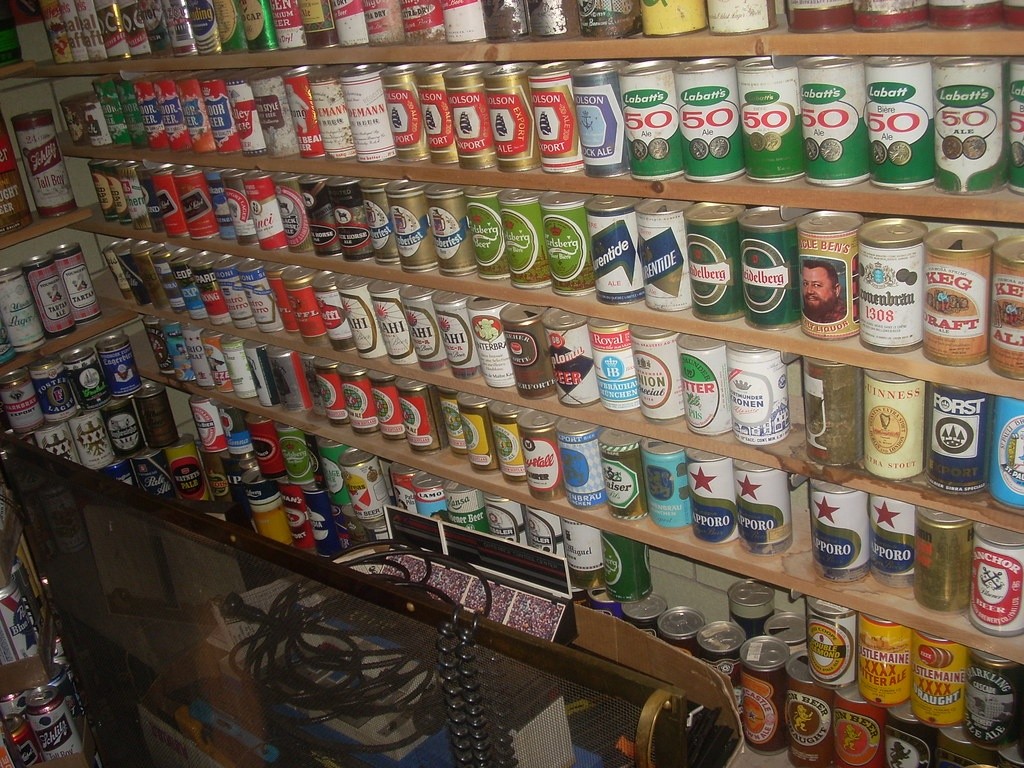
0,0,1024,768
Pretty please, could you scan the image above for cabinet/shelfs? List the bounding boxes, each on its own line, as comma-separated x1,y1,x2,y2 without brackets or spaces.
0,1,1024,768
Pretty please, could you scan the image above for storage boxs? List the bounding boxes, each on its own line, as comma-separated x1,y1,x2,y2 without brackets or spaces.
138,547,746,768
